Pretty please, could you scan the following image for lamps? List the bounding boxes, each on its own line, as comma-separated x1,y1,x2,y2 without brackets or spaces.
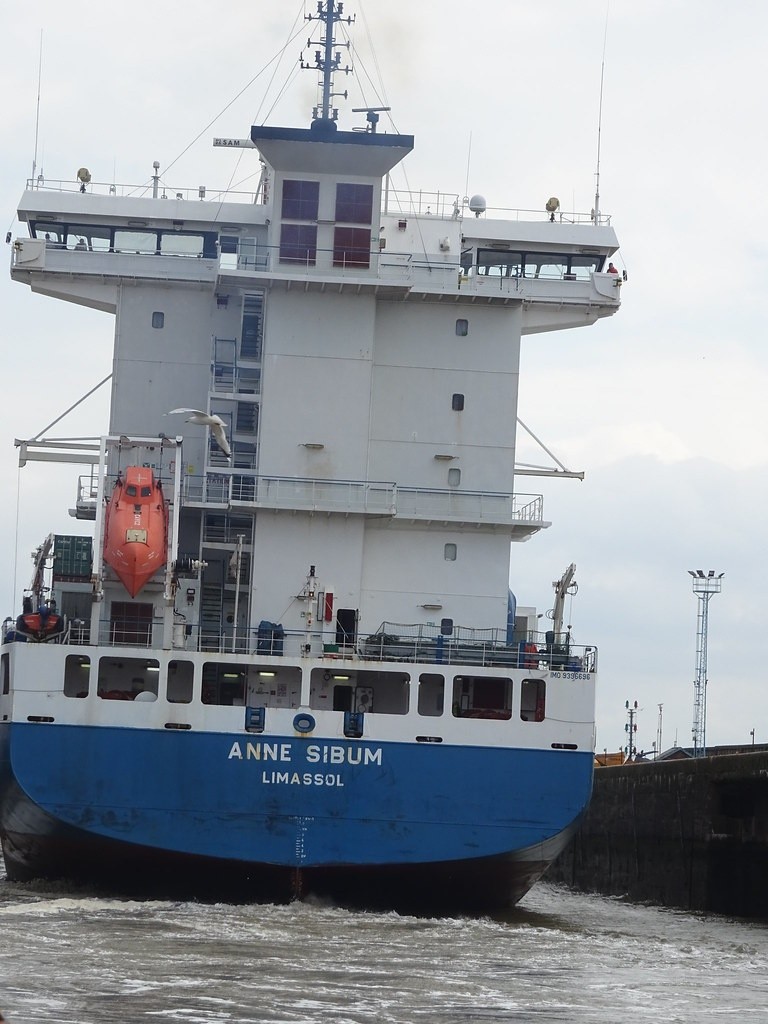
332,674,351,681
257,671,277,676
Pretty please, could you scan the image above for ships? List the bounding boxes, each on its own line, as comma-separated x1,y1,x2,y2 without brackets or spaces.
1,1,629,908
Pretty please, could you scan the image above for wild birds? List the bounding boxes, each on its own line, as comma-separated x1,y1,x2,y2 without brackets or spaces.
162,408,231,463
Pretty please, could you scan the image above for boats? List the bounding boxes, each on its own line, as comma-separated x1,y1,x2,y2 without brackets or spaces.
103,466,166,599
